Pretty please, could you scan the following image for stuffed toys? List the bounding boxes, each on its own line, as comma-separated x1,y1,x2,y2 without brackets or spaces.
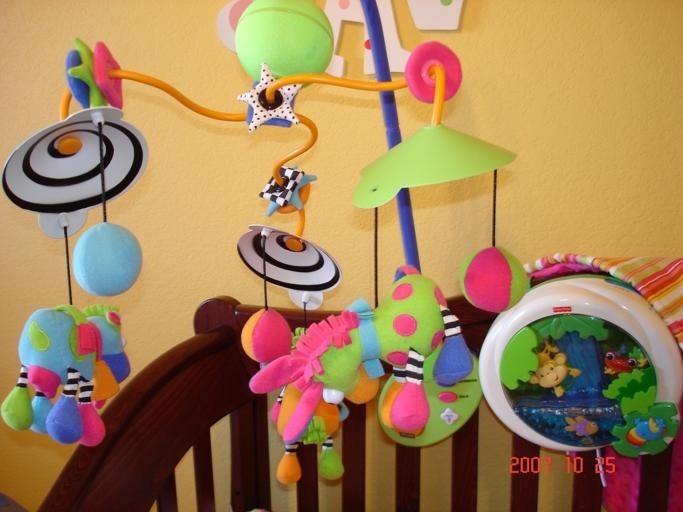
71,222,143,297
241,247,529,485
0,219,534,491
0,304,131,447
